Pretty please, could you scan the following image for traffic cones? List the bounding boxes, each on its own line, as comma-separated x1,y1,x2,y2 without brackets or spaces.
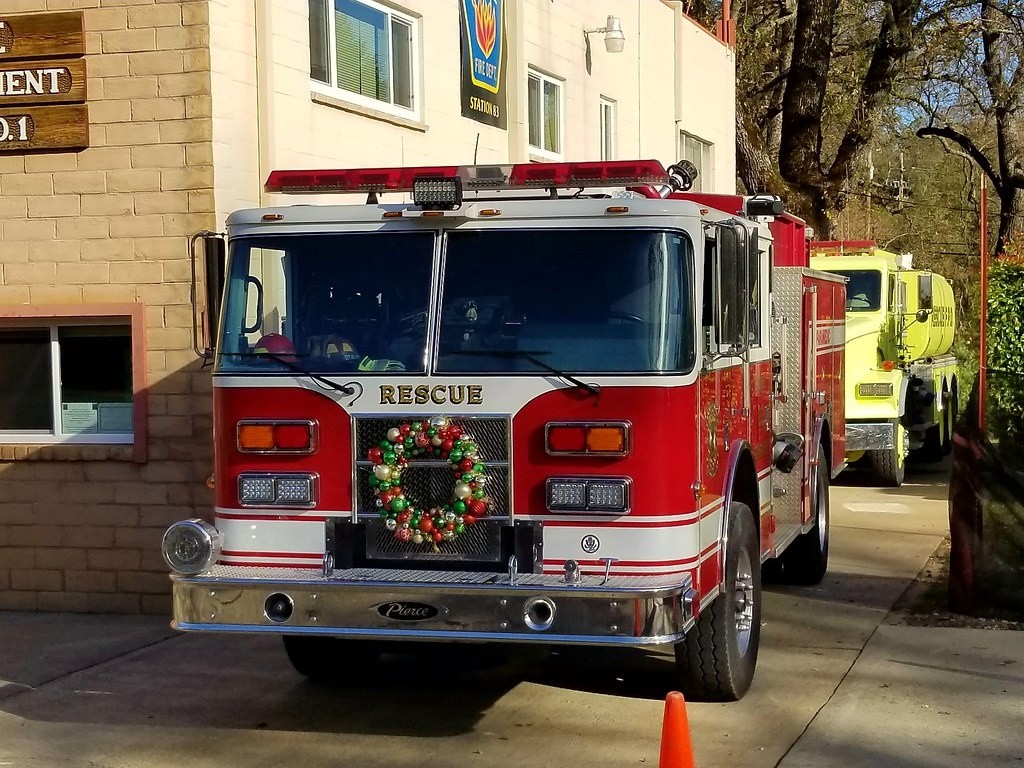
658,691,694,768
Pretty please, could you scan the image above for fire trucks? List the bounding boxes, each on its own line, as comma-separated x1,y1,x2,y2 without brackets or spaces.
160,158,846,701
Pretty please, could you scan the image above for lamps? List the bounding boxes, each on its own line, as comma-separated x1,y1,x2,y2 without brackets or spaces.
583,15,625,53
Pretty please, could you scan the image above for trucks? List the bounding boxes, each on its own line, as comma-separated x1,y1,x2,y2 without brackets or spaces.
810,242,959,486
